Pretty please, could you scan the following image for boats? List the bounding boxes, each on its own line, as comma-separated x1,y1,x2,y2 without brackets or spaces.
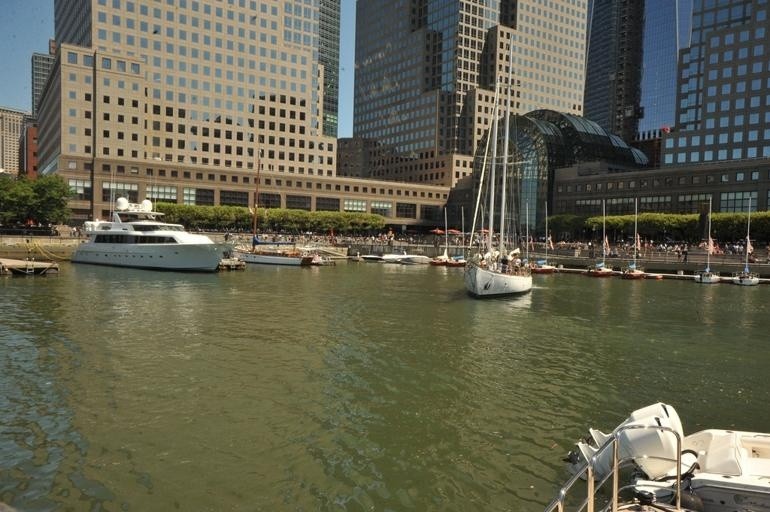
70,196,222,273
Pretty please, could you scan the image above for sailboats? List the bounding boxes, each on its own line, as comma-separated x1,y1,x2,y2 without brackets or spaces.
462,32,534,300
236,148,324,265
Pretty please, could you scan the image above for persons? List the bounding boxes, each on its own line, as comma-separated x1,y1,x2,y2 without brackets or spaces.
481,246,525,275
647,240,744,262
